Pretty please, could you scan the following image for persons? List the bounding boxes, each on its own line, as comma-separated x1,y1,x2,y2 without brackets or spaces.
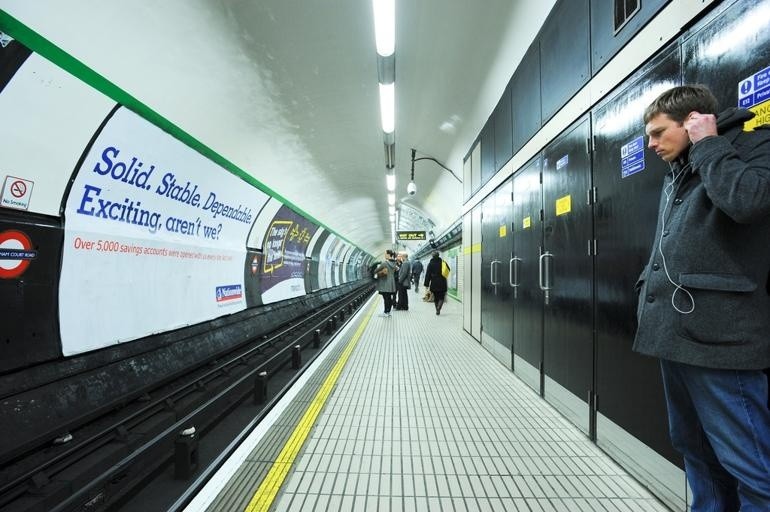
372,248,424,313
632,83,770,510
424,250,450,316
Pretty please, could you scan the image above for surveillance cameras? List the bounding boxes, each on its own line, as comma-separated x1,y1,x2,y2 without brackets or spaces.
407,182,417,196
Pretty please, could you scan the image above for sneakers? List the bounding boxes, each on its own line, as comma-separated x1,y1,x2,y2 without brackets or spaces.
378,311,392,317
436,306,440,314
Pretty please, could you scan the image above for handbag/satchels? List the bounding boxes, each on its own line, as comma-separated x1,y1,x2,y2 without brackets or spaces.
441,261,449,278
377,267,388,278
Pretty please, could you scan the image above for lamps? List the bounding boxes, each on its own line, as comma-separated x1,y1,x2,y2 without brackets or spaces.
372,0,396,245
